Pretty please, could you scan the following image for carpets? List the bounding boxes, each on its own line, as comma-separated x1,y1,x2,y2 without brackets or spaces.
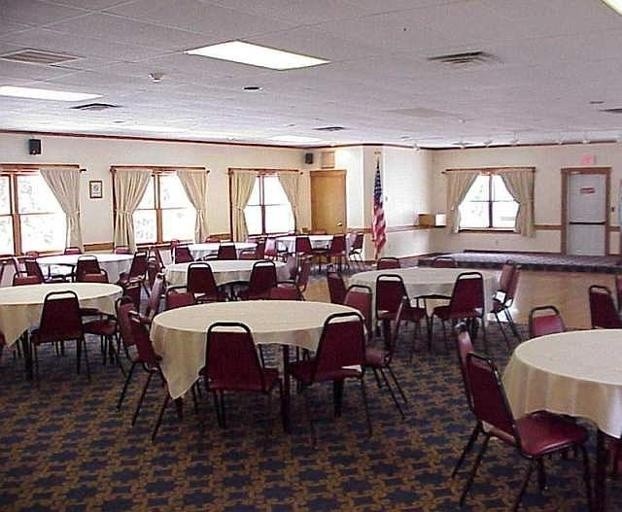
0,321,622,512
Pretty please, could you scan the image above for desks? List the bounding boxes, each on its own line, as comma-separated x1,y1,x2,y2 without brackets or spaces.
348,266,501,351
484,329,622,512
166,260,291,301
0,282,124,381
418,254,621,328
149,300,369,434
276,235,354,273
35,253,133,283
173,242,258,263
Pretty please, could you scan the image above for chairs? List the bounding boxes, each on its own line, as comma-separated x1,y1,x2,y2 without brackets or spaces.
429,272,488,356
186,263,225,302
267,280,306,301
217,240,237,260
365,296,407,417
286,312,373,445
348,231,364,272
127,310,221,441
119,251,148,313
115,296,159,409
76,255,109,283
146,256,162,291
588,285,622,329
81,268,108,284
175,244,194,264
430,256,457,268
490,260,522,352
63,246,82,256
166,286,198,310
294,237,331,274
326,272,348,304
238,234,268,260
319,233,350,274
141,272,166,325
236,260,277,301
23,257,45,280
377,257,401,268
199,322,283,454
78,281,142,372
149,246,164,268
375,274,430,356
295,258,312,292
529,305,566,339
341,284,372,341
459,352,593,512
112,245,130,253
206,236,220,243
31,291,92,385
12,271,42,287
450,322,503,480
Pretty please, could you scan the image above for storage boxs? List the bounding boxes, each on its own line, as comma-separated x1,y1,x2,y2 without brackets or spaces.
418,214,447,228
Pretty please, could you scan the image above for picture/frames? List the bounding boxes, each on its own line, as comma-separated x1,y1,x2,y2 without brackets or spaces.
89,179,103,199
